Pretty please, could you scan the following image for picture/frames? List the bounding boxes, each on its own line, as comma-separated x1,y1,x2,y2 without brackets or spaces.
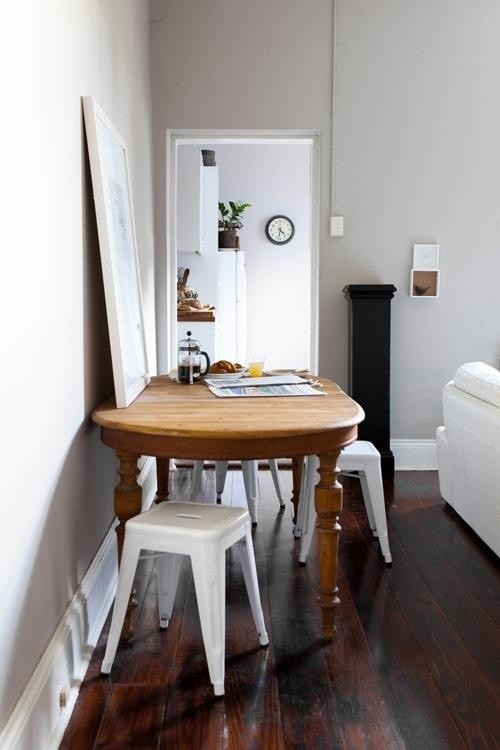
81,94,151,409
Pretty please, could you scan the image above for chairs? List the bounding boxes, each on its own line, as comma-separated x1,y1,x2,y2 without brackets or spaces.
435,360,500,560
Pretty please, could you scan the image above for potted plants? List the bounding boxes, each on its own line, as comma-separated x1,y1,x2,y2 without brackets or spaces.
218,200,251,248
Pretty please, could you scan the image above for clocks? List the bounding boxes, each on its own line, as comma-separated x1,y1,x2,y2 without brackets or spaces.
265,215,295,245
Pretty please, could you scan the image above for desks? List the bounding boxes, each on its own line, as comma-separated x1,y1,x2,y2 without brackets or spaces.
91,369,366,645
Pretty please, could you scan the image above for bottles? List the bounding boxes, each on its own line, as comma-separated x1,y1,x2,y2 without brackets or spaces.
176,330,210,384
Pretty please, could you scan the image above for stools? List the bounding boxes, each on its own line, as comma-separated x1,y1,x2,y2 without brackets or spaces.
293,440,393,568
100,500,270,697
215,457,285,527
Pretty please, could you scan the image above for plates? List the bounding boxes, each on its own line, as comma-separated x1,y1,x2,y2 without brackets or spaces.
203,365,249,380
264,369,311,376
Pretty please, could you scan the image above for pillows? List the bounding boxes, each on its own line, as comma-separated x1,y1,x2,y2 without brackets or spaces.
452,357,500,408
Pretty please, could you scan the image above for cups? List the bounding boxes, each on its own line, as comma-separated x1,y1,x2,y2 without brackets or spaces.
250,357,264,378
